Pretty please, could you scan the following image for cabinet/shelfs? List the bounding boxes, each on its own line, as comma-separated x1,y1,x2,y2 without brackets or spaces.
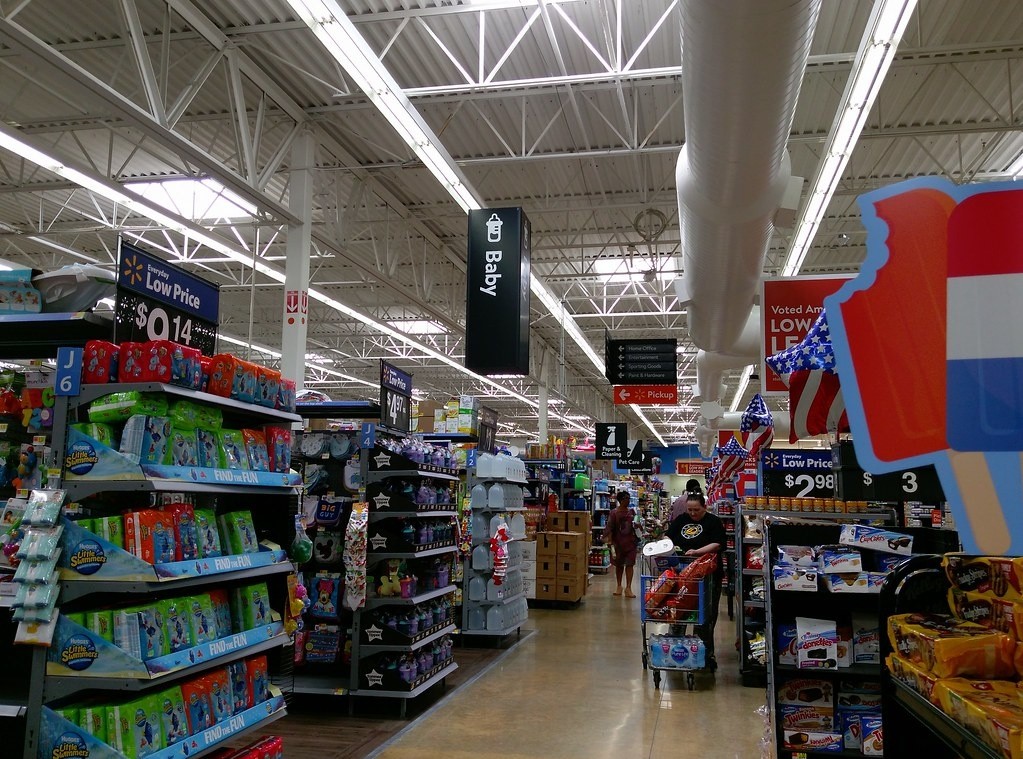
412,432,529,646
523,460,594,515
715,502,736,597
0,312,303,759
761,521,958,759
574,491,612,574
735,503,901,688
269,399,462,724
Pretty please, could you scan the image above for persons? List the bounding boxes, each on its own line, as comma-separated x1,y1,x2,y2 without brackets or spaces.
664,487,728,672
607,491,637,598
672,479,700,522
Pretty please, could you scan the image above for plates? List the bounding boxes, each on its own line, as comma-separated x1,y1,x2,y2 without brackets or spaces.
292,433,350,491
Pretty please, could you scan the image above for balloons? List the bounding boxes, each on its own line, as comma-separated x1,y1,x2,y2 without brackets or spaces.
740,393,775,460
764,307,851,444
704,436,749,499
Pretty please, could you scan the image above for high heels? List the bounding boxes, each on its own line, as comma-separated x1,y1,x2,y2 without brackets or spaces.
626,588,636,598
613,586,623,595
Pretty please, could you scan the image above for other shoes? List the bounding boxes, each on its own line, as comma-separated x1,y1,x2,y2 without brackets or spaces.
703,655,717,672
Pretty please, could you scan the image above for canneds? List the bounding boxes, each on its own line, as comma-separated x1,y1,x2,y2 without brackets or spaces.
745,496,867,513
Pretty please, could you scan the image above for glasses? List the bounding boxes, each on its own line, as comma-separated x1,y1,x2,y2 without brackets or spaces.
688,492,701,495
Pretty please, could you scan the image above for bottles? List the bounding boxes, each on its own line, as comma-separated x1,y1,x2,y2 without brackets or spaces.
649,633,706,669
568,456,613,567
466,446,560,632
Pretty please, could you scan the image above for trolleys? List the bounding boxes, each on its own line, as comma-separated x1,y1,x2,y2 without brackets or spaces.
639,555,715,690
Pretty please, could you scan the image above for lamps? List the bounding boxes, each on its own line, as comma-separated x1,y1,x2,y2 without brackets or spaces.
0,0,918,448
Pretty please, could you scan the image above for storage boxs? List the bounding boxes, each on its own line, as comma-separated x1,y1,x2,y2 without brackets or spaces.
0,338,915,759
